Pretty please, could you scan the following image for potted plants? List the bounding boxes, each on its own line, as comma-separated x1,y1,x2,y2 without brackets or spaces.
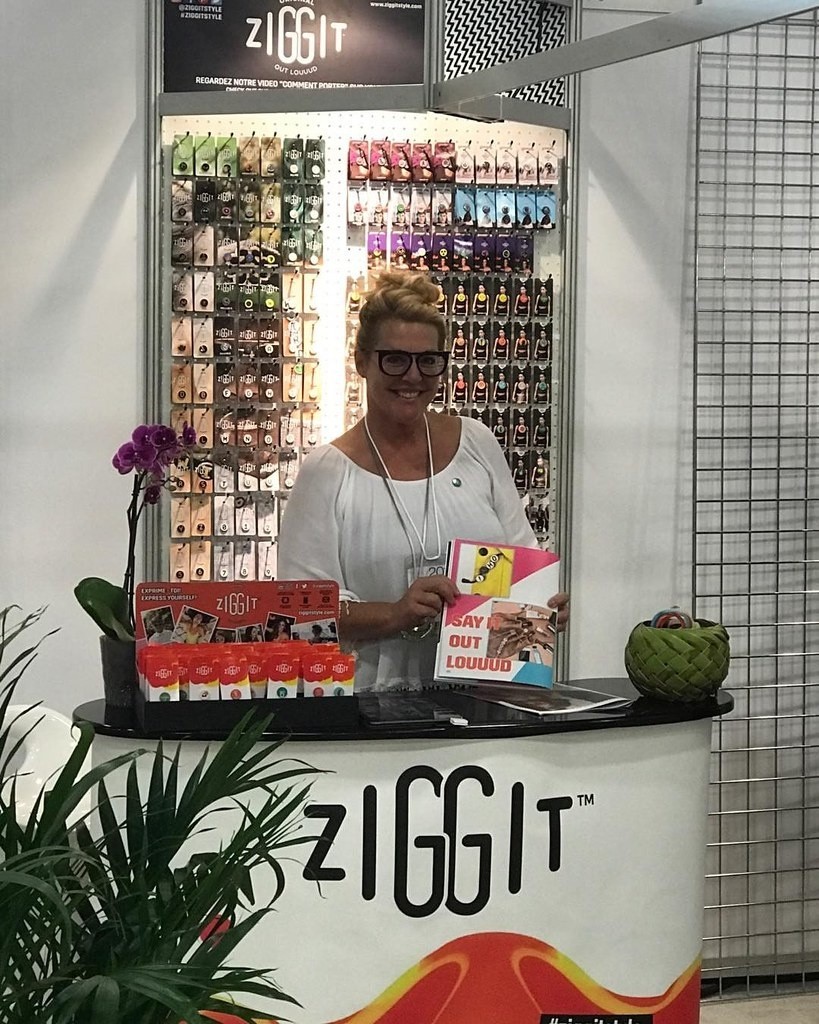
74,421,196,709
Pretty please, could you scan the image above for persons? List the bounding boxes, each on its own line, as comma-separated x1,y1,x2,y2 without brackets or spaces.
350,203,556,532
275,274,571,694
140,606,339,646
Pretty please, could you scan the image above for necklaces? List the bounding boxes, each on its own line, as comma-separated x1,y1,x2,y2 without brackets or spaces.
363,414,434,643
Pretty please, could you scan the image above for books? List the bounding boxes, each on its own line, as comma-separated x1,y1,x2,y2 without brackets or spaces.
432,538,562,692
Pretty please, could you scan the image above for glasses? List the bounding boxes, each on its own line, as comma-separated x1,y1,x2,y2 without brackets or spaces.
369,349,452,378
